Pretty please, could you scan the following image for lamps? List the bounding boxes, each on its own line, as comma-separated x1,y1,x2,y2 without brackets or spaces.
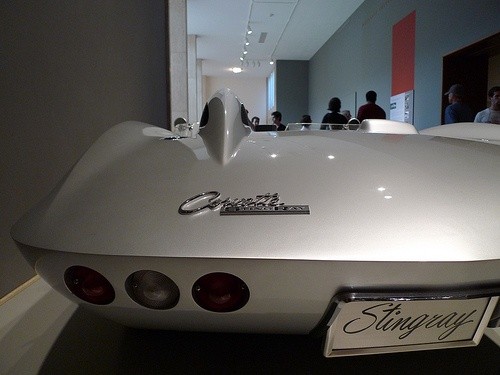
240,26,252,61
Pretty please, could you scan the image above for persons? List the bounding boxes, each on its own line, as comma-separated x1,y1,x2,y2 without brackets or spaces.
320,96,348,130
440,83,473,125
356,90,386,123
299,114,312,131
341,109,352,121
474,86,500,125
251,116,259,126
271,111,286,131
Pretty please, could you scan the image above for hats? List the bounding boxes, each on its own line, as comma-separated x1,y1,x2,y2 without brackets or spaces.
444,84,465,96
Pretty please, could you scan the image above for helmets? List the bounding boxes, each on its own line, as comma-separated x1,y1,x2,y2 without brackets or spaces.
327,97,341,111
